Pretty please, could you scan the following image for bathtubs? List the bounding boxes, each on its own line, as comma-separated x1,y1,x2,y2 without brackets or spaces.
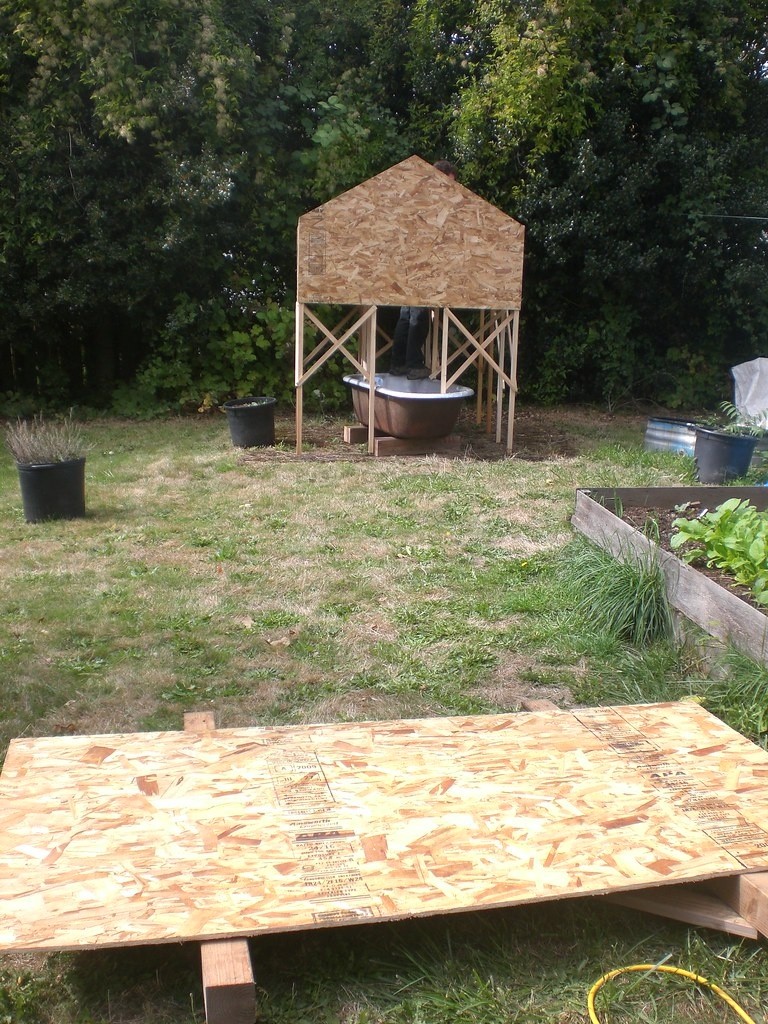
342,373,475,440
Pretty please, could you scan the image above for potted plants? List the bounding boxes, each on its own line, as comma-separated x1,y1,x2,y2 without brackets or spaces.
222,396,278,451
691,400,768,486
0,403,98,526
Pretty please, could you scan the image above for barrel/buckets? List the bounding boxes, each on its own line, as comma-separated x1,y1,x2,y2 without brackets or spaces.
17,457,86,523
696,425,759,484
223,396,276,448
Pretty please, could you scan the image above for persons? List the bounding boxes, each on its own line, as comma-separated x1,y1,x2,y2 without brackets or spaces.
390,306,432,381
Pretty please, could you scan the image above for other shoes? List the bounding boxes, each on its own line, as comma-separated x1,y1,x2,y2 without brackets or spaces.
407,366,434,380
388,360,407,375
